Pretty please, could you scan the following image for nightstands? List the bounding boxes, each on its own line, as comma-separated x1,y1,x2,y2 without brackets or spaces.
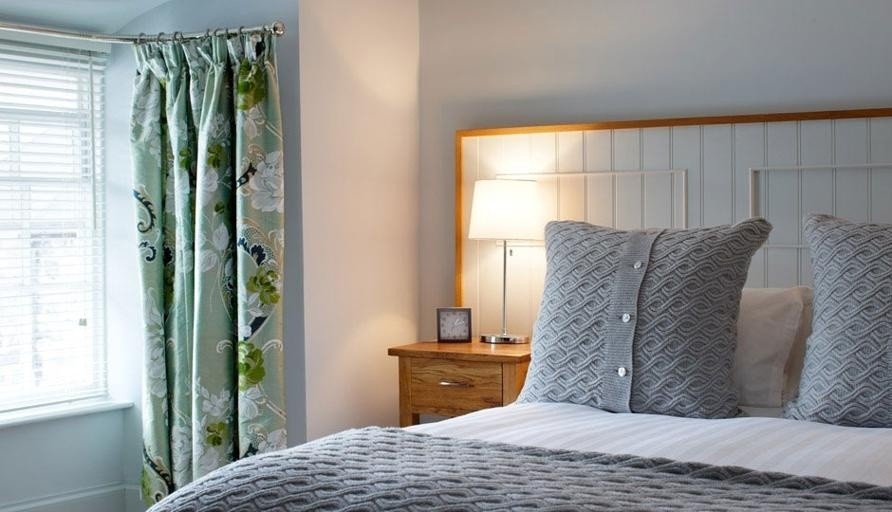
386,342,531,426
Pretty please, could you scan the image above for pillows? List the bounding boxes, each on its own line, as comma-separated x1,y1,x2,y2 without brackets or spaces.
513,215,776,420
783,212,891,428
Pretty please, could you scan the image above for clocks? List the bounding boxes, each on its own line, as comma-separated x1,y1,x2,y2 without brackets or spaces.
437,307,472,343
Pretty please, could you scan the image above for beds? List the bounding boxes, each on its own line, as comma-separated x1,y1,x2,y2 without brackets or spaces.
142,402,892,512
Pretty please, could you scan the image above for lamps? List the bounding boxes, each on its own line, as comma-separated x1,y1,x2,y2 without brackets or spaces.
468,180,532,345
